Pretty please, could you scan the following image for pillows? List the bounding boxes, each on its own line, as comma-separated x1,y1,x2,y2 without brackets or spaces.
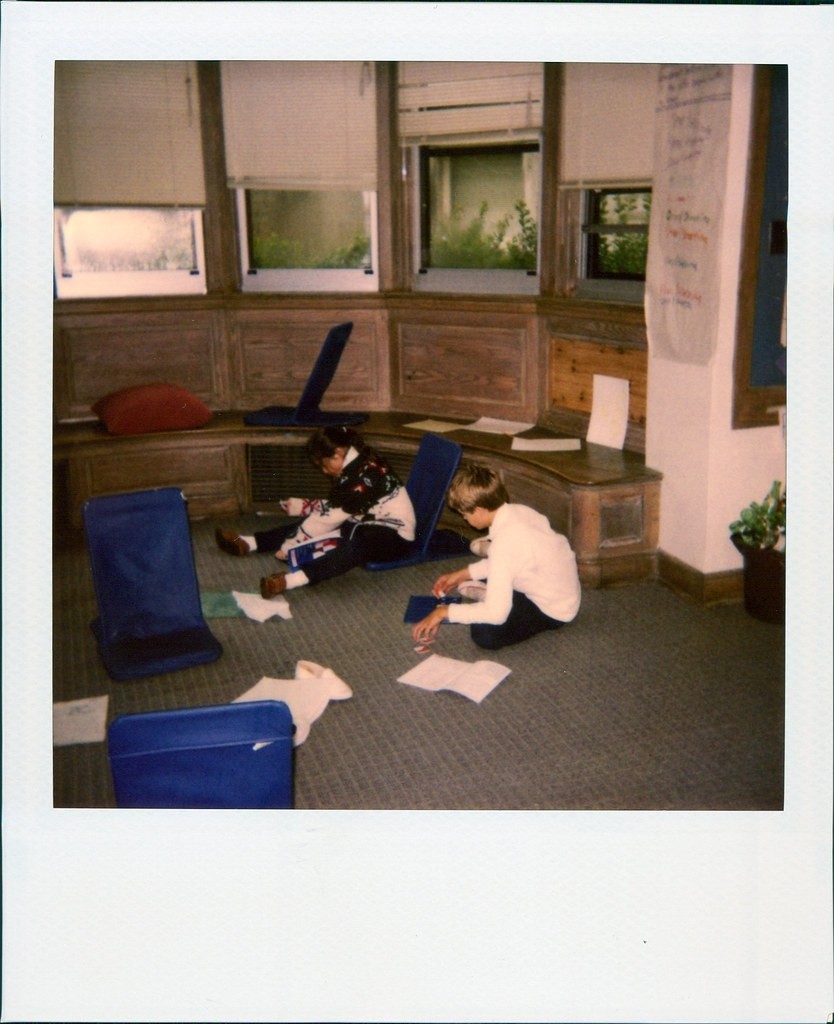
93,382,212,435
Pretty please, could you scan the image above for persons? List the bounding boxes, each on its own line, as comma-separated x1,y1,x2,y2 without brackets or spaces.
412,463,583,648
216,426,417,599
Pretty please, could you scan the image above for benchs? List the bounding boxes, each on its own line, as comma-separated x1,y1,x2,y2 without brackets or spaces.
52,409,665,589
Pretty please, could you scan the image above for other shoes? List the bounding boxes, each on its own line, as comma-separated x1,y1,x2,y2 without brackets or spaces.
259,571,289,599
215,526,249,556
458,579,487,602
469,536,492,556
